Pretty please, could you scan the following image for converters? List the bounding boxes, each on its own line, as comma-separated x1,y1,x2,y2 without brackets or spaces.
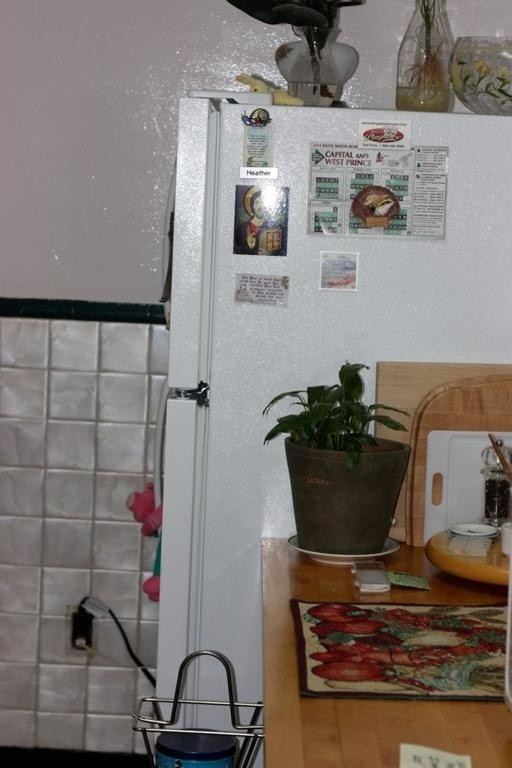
72,613,93,649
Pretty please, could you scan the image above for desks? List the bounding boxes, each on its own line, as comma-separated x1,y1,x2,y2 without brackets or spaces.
260,536,512,767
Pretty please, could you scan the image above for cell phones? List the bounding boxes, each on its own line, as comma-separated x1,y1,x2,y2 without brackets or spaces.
354,561,390,591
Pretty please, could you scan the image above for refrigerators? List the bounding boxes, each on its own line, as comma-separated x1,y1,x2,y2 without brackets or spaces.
149,86,512,755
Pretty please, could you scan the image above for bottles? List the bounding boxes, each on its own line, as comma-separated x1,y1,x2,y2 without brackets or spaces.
480,441,512,528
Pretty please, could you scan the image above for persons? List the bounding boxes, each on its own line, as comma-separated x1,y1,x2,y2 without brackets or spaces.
234,186,286,256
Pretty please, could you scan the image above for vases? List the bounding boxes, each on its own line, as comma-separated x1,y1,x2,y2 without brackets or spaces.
447,35,512,115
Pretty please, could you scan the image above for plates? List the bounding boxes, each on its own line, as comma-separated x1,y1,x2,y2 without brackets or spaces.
446,522,500,539
286,531,403,567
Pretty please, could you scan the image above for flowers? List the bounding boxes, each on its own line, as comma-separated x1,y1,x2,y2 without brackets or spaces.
451,47,511,111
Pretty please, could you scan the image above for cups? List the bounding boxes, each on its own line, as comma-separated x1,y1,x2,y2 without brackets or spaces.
500,521,511,559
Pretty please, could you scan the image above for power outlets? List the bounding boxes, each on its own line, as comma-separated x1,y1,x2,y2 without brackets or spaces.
69,602,98,651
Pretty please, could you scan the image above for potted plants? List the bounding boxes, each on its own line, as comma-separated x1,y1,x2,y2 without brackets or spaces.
226,0,367,108
260,356,416,556
395,0,454,113
